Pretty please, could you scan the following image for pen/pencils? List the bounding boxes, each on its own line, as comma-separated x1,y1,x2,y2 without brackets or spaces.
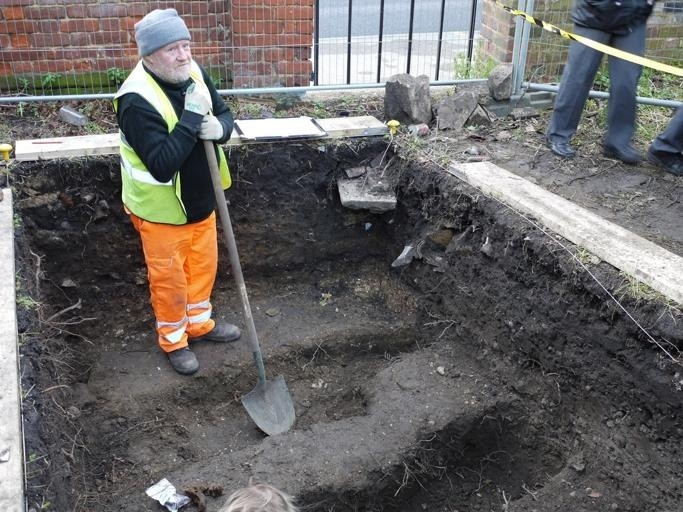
32,142,62,144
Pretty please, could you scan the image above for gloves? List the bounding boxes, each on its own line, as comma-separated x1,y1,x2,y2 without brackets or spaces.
184,82,224,140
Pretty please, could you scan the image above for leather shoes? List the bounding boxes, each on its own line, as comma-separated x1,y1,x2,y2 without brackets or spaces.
602,144,683,177
546,133,575,157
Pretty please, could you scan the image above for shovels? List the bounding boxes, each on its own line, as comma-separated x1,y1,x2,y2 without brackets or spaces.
204,140,296,435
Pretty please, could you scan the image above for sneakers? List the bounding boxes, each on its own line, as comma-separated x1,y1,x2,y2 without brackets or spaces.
167,322,241,375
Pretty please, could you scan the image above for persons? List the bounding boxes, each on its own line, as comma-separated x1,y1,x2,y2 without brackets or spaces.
218,483,298,512
545,0,683,176
113,8,241,375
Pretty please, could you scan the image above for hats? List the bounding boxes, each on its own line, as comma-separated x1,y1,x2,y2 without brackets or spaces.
134,8,191,57
